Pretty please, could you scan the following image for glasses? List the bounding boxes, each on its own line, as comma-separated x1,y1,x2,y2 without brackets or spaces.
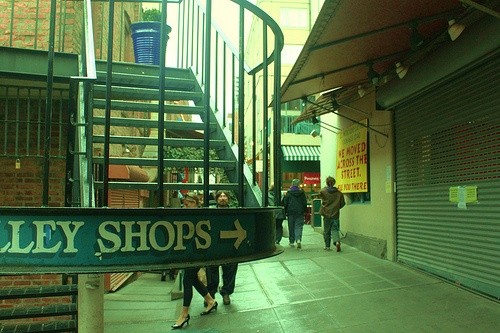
182,193,196,200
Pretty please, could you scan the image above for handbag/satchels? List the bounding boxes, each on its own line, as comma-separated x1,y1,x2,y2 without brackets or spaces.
282,219,290,238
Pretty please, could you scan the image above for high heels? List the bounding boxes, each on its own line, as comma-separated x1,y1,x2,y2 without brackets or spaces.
200,302,218,315
171,314,190,329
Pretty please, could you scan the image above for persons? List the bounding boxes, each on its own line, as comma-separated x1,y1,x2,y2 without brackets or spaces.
318,176,345,252
270,179,307,248
204,190,238,306
172,192,217,328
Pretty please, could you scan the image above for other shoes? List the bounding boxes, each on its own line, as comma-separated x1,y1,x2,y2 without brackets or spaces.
204,296,215,307
223,294,230,304
169,274,175,279
336,242,340,252
324,246,330,251
296,239,301,248
161,271,167,281
290,243,295,248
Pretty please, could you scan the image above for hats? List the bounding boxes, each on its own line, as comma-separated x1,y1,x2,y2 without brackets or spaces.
292,178,300,186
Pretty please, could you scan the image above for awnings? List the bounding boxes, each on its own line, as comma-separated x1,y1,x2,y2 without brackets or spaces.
281,145,320,161
267,0,500,124
